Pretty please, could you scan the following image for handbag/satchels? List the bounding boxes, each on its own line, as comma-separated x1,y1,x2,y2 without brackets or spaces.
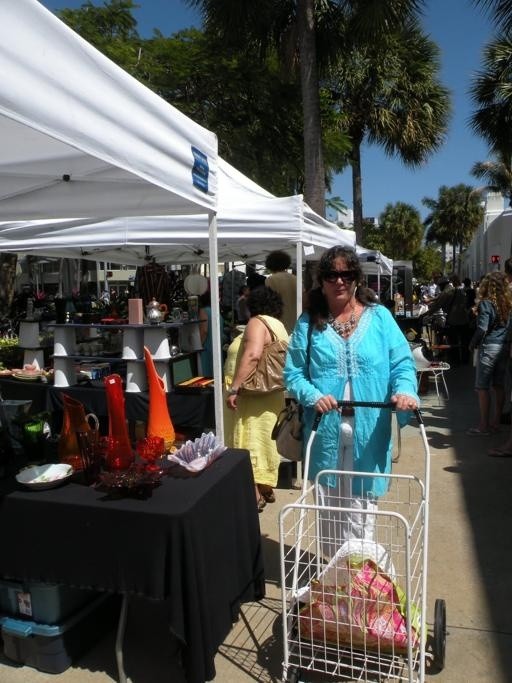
239,340,288,396
275,401,304,462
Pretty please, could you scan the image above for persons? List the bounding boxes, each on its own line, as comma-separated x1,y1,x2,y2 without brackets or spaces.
223,286,290,510
464,271,510,436
370,270,483,367
100,289,110,304
283,245,421,562
110,286,116,300
35,286,45,299
265,250,310,336
234,286,252,324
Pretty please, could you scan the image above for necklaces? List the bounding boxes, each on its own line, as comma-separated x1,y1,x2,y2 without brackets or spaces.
327,306,357,339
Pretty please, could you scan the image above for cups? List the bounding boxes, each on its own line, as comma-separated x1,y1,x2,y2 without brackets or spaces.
102,435,133,489
72,341,105,356
134,435,168,484
172,307,183,322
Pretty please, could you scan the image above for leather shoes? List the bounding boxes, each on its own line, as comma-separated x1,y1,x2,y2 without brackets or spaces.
256,487,275,510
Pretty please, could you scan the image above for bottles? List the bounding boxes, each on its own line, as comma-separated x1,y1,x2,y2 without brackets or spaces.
143,346,176,448
105,374,134,468
188,296,199,321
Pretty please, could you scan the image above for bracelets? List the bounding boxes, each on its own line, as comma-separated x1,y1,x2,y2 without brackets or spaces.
227,388,238,395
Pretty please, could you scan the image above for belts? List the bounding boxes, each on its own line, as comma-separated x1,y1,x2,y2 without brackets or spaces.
341,408,354,416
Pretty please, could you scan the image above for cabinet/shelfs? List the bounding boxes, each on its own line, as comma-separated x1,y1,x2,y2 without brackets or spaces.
13,315,104,350
46,319,207,394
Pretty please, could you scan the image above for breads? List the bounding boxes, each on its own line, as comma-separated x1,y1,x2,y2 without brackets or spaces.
22,365,38,377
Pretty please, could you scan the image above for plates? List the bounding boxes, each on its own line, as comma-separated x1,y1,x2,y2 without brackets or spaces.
13,371,41,382
183,274,208,297
15,461,74,490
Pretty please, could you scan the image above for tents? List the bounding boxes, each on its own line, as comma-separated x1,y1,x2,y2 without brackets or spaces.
302,248,393,303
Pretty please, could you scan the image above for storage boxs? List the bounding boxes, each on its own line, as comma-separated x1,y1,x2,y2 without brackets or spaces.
5,578,80,624
0,594,119,675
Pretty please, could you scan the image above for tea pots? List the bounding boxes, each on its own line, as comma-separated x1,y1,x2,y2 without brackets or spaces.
145,296,168,325
61,391,99,481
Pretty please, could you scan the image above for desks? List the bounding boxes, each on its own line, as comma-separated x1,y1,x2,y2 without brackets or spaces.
0,449,266,683
0,375,214,430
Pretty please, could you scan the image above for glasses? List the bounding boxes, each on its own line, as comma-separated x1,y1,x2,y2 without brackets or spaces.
323,271,354,285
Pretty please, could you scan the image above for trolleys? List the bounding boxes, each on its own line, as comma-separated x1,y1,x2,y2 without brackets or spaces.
277,397,450,682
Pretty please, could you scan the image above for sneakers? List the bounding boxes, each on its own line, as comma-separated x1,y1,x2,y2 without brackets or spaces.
466,427,488,436
488,447,511,456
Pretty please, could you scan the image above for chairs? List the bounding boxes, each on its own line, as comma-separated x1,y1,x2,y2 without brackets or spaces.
412,345,451,401
